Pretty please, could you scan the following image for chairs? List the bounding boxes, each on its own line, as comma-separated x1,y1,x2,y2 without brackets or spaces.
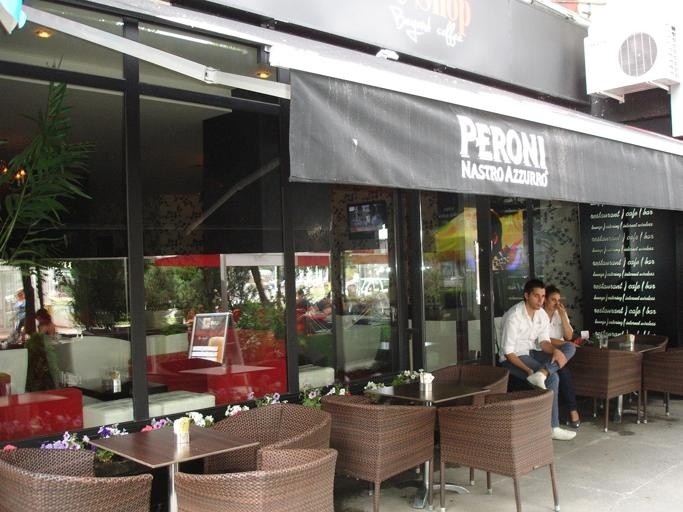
321,395,436,512
174,448,338,512
429,364,510,485
438,389,560,512
608,335,668,407
343,325,382,385
644,348,683,423
567,348,643,432
209,403,332,473
0,449,153,512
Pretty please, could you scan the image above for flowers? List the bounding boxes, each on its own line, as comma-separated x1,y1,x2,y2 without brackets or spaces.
324,384,351,396
298,383,322,407
137,416,173,432
224,404,250,417
362,381,385,403
389,368,424,386
85,423,128,461
37,432,84,449
245,391,288,407
186,412,214,427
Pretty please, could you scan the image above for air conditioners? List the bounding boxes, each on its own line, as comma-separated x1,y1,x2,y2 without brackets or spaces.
583,20,679,103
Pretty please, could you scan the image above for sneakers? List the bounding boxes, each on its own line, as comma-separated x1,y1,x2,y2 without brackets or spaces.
527,372,546,388
552,427,575,440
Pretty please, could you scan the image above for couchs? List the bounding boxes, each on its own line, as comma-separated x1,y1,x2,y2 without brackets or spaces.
128,330,287,407
0,373,83,442
299,363,334,391
83,391,215,428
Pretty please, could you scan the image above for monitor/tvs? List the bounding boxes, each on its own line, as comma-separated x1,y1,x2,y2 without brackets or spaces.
347,200,385,240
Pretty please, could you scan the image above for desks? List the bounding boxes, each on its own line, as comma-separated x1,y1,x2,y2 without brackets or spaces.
87,424,259,511
581,343,657,424
364,382,491,508
179,366,276,403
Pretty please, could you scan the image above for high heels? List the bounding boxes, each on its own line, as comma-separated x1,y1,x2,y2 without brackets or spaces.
572,420,580,427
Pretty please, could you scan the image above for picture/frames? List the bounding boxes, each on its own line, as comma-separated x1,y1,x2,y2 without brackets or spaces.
188,312,229,364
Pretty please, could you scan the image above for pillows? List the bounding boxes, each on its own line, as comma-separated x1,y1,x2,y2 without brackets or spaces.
344,359,389,379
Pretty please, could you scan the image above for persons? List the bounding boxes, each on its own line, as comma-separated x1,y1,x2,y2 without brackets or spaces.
34,308,55,336
293,285,368,333
13,288,30,344
499,279,576,441
543,284,582,427
